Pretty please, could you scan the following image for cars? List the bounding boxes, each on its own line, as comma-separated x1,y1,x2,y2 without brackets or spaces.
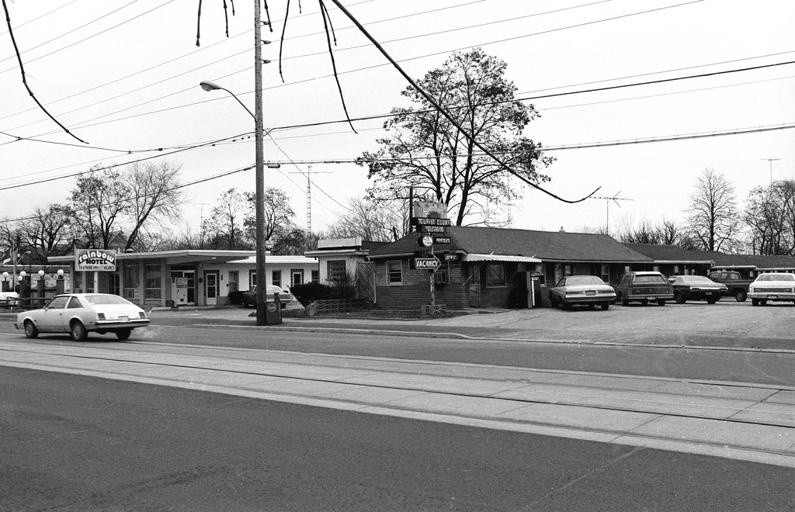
610,269,676,307
12,290,152,343
241,283,294,310
663,273,730,305
546,273,619,313
745,272,795,308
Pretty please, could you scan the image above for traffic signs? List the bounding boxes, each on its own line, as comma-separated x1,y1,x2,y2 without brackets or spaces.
415,255,443,272
415,225,446,234
412,217,451,227
434,237,450,245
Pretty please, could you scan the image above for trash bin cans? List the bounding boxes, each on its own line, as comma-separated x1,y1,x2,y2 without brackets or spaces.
264,299,282,324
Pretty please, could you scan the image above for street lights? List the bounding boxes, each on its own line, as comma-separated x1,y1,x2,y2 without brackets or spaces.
37,270,45,306
198,78,266,327
19,270,27,305
56,268,64,294
12,250,32,291
1,271,11,291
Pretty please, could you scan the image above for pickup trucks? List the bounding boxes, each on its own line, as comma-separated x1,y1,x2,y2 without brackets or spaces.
708,269,755,303
